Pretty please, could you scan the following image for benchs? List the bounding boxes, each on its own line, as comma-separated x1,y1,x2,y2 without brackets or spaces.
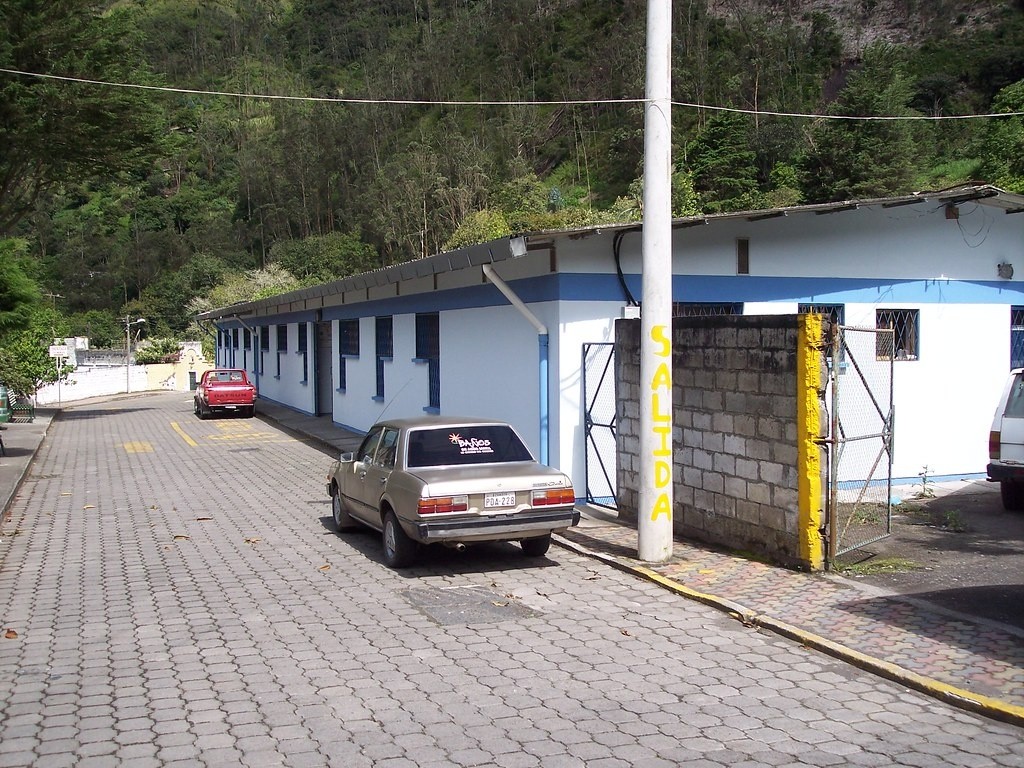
8,389,35,422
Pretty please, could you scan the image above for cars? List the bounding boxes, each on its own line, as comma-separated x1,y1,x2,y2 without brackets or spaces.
327,417,580,568
0,386,17,422
986,368,1024,511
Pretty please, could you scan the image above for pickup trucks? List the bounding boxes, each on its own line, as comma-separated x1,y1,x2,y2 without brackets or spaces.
194,369,257,419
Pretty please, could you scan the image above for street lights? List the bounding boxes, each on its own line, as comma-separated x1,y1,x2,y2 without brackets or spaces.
126,314,145,393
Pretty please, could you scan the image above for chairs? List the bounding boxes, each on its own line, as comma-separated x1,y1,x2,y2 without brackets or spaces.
211,376,218,381
391,442,424,467
